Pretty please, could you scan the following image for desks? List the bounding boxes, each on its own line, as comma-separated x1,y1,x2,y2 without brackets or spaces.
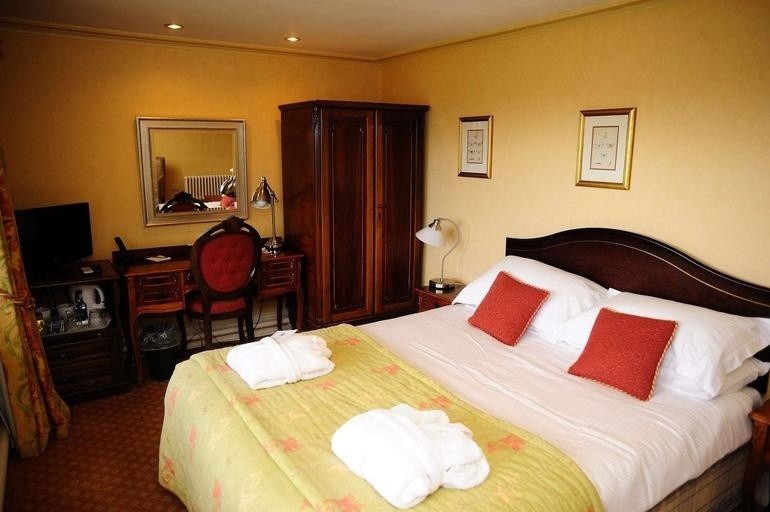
112,237,305,387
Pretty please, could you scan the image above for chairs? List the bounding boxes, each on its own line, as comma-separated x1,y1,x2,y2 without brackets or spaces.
177,215,261,350
160,191,208,212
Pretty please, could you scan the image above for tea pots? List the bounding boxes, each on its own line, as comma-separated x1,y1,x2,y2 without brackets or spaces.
69,285,104,309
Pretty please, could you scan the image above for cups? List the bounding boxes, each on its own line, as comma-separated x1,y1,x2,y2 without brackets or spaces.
57,303,75,317
35,306,51,320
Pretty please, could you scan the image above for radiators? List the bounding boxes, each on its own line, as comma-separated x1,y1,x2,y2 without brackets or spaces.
185,175,231,200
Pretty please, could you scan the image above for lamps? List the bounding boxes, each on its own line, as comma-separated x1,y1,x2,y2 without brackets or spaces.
416,218,460,290
251,176,285,250
221,176,235,197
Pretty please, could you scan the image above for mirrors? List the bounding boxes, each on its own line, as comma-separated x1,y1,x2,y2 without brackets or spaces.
135,116,249,227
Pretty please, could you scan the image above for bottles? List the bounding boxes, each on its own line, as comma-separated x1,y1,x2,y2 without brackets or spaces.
75,297,88,321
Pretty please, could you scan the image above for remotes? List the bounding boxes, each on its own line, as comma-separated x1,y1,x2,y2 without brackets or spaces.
81,266,93,275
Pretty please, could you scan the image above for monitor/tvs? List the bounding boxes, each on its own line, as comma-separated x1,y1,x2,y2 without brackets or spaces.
15,202,93,281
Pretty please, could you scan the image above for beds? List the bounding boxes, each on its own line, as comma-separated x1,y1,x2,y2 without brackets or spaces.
158,227,770,512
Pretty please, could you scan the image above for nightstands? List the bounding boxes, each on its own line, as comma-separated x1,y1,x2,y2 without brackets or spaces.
414,286,462,311
744,399,769,507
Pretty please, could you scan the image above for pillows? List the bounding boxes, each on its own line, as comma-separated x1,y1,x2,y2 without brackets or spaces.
451,256,770,403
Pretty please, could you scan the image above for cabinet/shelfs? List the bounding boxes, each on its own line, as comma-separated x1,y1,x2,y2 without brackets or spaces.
29,260,130,401
278,100,428,322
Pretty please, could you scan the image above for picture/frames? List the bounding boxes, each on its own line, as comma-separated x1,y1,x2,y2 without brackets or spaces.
457,115,493,177
575,106,636,191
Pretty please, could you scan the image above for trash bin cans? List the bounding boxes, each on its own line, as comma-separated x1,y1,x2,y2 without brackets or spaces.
142,320,187,382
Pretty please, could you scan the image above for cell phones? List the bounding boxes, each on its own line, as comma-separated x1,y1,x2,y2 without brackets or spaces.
94,264,100,273
